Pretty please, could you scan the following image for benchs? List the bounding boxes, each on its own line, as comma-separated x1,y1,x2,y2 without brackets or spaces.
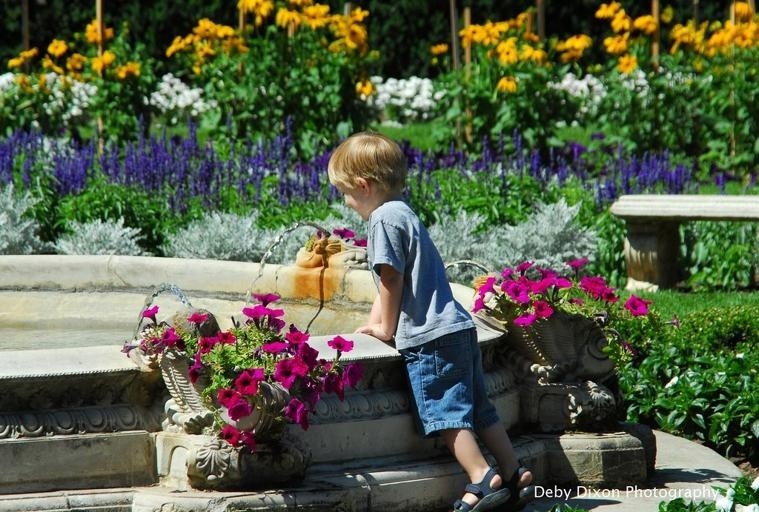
606,191,758,294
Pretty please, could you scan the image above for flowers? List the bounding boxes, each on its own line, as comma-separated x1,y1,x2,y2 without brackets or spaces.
120,291,366,454
469,256,652,329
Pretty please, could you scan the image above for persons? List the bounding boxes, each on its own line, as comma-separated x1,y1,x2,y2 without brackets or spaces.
327,133,541,511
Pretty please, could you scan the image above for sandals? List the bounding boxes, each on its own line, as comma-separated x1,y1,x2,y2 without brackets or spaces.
454,465,535,512
